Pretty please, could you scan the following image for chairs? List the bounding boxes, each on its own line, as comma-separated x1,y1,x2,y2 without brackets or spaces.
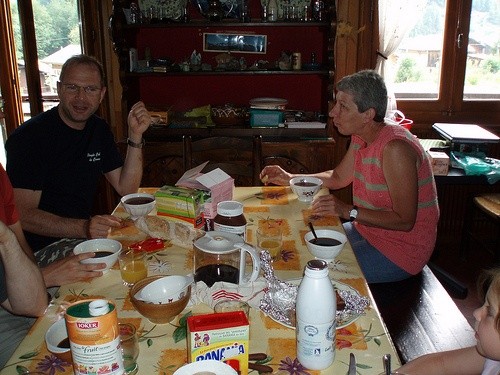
183,134,263,186
460,192,500,265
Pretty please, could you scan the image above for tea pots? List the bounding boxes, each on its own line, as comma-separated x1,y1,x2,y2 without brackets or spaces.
197,0,234,24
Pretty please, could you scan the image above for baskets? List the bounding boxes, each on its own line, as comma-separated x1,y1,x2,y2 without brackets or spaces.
211,105,251,128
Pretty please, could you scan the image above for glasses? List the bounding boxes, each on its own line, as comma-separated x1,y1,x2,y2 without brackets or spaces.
61,82,103,96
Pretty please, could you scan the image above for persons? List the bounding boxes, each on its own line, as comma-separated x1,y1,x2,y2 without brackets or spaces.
260,70,441,284
5,54,151,265
394,269,500,375
0,165,106,374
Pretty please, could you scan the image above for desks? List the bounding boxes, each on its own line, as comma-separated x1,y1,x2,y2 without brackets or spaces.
0,186,403,375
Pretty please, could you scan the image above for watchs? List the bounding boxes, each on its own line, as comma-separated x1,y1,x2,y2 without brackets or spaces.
349,206,358,222
127,138,145,148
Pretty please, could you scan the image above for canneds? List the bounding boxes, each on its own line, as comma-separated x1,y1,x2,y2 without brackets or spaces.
129,48,137,71
293,52,301,70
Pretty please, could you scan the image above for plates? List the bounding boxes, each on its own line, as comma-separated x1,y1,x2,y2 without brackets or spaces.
267,277,362,330
249,97,288,106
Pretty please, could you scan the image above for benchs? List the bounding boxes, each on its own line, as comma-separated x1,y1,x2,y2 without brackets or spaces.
383,263,478,362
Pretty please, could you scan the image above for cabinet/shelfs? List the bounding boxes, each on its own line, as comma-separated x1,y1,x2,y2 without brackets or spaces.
418,139,495,263
109,0,337,188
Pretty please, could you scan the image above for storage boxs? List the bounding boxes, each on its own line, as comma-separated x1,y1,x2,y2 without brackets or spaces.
427,151,449,176
154,160,235,230
186,311,249,375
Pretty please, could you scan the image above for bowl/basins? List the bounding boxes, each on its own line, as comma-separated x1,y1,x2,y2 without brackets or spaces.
396,117,413,131
120,193,157,221
129,274,194,325
289,176,323,201
73,239,123,273
173,359,238,375
44,317,73,363
304,229,348,263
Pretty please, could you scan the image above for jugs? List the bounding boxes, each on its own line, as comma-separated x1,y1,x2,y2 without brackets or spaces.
191,231,261,288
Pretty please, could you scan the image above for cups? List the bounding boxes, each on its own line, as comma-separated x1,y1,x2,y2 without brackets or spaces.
118,322,140,375
256,226,282,262
292,52,301,70
150,114,161,124
283,5,313,23
142,5,192,24
117,248,148,286
213,299,250,321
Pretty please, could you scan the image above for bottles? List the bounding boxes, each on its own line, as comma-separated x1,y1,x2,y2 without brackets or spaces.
159,0,166,24
267,0,278,22
130,3,138,24
313,0,325,22
295,259,335,372
224,50,245,72
212,200,247,242
